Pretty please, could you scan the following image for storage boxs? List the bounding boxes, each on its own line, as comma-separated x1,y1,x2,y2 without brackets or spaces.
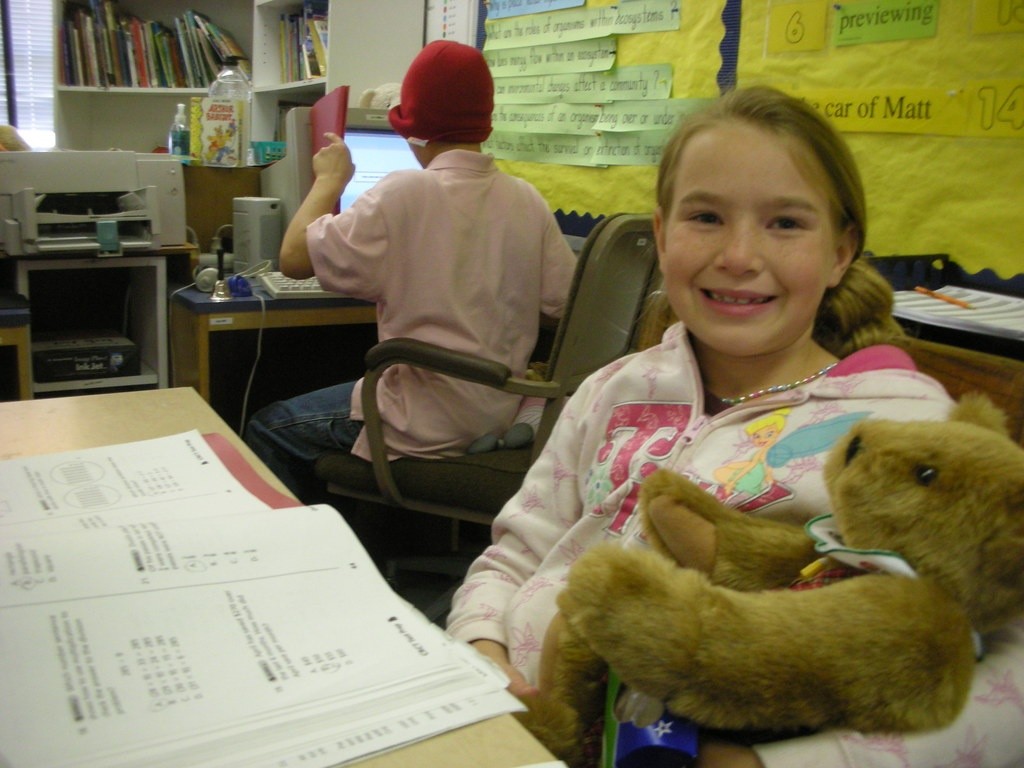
31,305,139,382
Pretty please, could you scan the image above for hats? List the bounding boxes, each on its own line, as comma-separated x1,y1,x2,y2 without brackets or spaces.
387,39,494,144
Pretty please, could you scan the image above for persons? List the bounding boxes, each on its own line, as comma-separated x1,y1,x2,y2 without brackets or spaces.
244,40,579,498
447,83,1024,768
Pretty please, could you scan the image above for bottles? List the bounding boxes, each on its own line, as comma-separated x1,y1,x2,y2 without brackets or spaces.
167,103,190,156
205,56,252,169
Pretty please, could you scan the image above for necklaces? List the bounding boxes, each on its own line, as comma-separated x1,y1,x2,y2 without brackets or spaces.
718,361,839,407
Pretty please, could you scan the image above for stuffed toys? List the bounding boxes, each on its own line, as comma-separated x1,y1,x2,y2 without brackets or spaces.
510,390,1024,766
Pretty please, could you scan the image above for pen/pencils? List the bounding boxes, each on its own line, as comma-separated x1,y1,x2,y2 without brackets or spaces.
915,286,977,310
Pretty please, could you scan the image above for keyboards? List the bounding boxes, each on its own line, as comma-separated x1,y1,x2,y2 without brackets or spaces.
258,271,352,299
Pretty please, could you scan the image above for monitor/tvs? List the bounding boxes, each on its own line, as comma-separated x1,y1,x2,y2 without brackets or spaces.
260,107,422,248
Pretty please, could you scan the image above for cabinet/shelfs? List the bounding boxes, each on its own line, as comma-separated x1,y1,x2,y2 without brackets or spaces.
49,0,249,168
248,0,427,169
17,250,167,399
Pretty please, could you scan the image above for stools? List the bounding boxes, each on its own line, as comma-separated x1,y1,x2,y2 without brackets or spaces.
0,294,36,401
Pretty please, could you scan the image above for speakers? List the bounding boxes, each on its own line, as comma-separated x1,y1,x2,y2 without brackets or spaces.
233,197,283,275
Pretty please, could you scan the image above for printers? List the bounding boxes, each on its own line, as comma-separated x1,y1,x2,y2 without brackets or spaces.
0,150,186,258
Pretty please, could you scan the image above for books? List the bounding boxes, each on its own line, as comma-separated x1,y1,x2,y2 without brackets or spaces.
278,2,328,84
888,284,1024,343
310,85,351,216
3,428,531,768
59,0,248,90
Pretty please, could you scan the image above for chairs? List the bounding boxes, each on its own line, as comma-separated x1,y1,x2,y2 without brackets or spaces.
311,211,668,624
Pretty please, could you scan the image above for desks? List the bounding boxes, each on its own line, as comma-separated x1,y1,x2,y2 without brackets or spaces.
166,267,381,405
0,385,569,768
